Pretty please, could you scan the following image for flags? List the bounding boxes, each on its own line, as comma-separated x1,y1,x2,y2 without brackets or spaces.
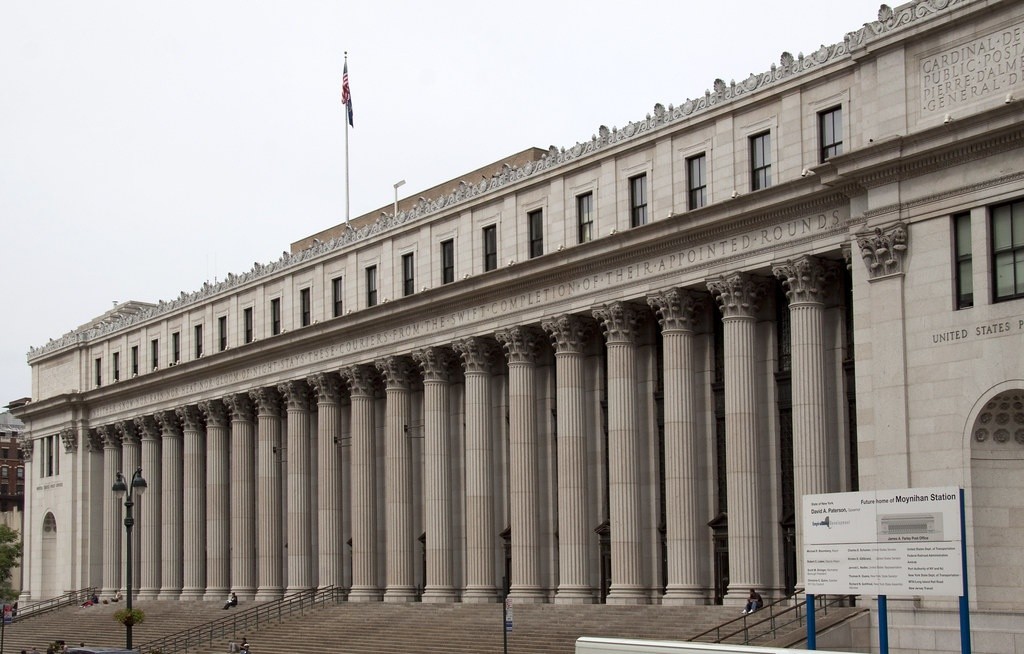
340,61,355,128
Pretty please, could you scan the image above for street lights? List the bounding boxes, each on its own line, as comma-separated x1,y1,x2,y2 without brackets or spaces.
111,465,148,649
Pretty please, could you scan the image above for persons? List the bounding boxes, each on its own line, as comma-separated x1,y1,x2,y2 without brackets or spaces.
222,592,238,611
79,592,99,609
227,637,252,654
741,587,764,615
20,640,94,654
111,589,124,603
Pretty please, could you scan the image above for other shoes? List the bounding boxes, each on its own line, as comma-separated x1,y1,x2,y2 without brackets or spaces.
748,609,753,613
742,609,747,614
